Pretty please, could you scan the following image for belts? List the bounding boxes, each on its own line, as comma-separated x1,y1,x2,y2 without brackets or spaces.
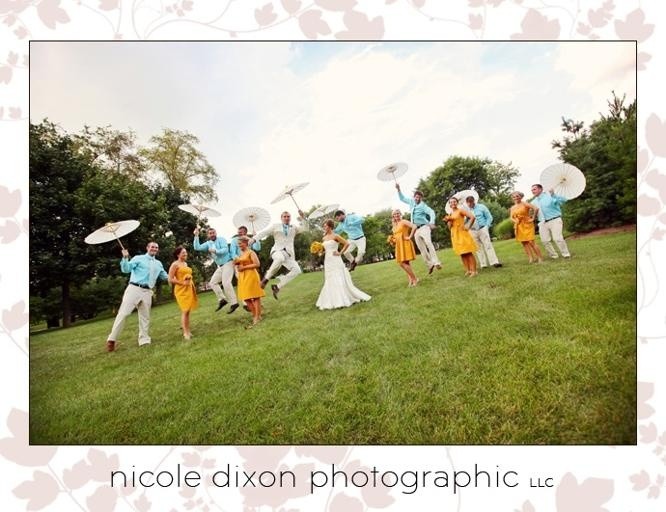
472,226,484,231
545,216,562,223
130,281,151,290
219,258,234,267
353,236,363,241
417,224,425,229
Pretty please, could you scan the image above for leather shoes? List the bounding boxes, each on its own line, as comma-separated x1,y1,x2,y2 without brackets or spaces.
106,341,116,352
494,263,502,268
270,283,280,300
214,299,227,312
227,303,239,314
428,265,435,274
349,260,358,272
408,278,420,287
261,278,269,289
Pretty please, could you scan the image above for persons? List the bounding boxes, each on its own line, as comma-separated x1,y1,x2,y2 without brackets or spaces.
315,218,372,311
530,184,571,261
333,210,366,272
390,209,420,288
193,227,240,314
447,197,478,278
463,196,502,268
167,245,199,339
395,183,442,275
248,208,303,300
509,190,544,264
107,241,170,352
237,236,266,325
230,225,261,312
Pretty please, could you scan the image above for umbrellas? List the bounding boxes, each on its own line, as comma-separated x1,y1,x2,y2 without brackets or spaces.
84,219,140,250
177,203,221,229
308,203,340,221
376,161,409,183
540,162,587,201
269,181,310,209
232,205,271,232
444,189,480,214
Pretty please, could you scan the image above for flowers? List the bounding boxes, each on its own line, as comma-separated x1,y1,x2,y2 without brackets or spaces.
512,207,524,229
443,214,454,227
387,235,396,248
178,266,193,282
310,241,323,257
232,255,249,268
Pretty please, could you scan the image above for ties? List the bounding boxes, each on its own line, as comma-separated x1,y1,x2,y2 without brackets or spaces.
470,208,479,230
538,197,546,225
148,259,155,289
411,204,416,224
210,244,217,258
285,224,288,237
